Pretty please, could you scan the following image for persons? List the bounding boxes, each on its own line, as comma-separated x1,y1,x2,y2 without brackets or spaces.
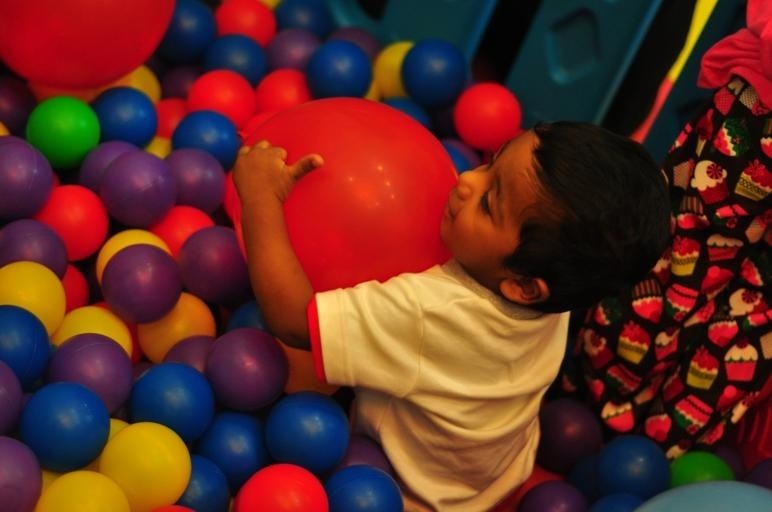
559,1,771,484
231,118,670,511
